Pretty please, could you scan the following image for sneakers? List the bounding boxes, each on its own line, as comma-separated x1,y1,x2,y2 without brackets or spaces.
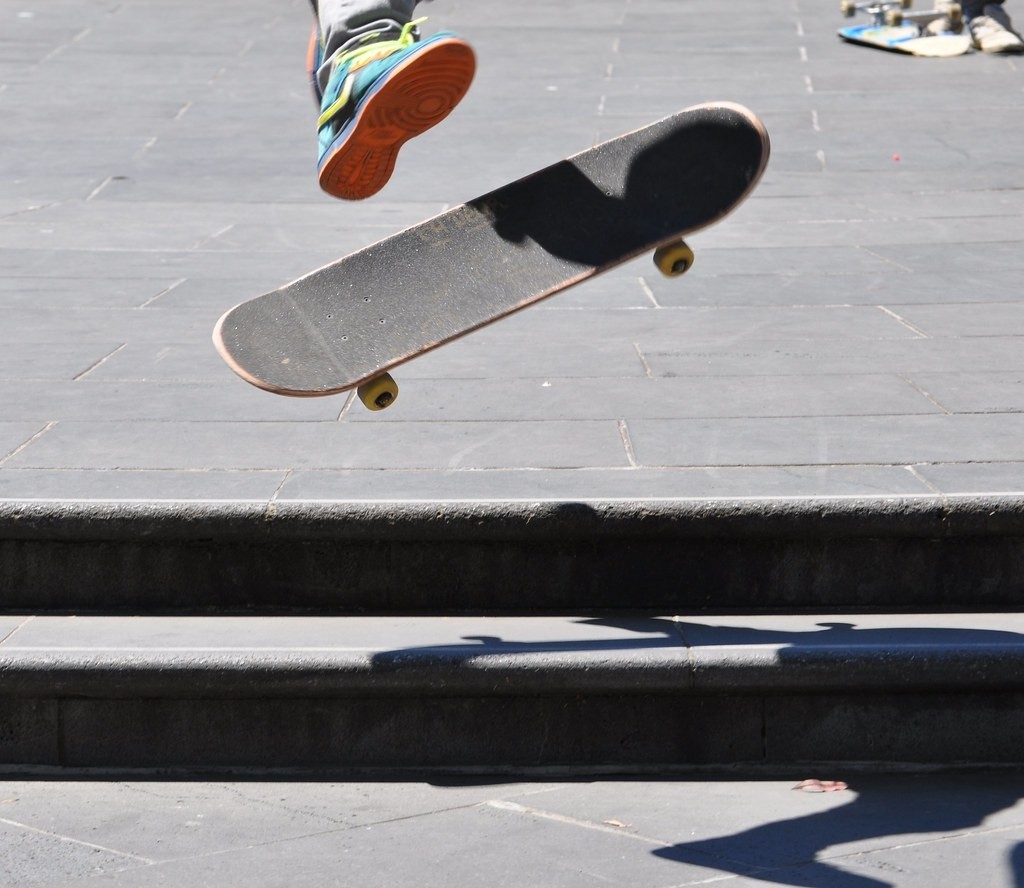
964,15,1024,55
316,10,477,198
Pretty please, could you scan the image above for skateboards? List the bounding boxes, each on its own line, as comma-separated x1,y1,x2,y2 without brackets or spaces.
212,99,772,412
836,1,972,58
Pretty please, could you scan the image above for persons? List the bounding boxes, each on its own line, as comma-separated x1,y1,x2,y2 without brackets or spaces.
305,1,478,201
928,0,1024,55
